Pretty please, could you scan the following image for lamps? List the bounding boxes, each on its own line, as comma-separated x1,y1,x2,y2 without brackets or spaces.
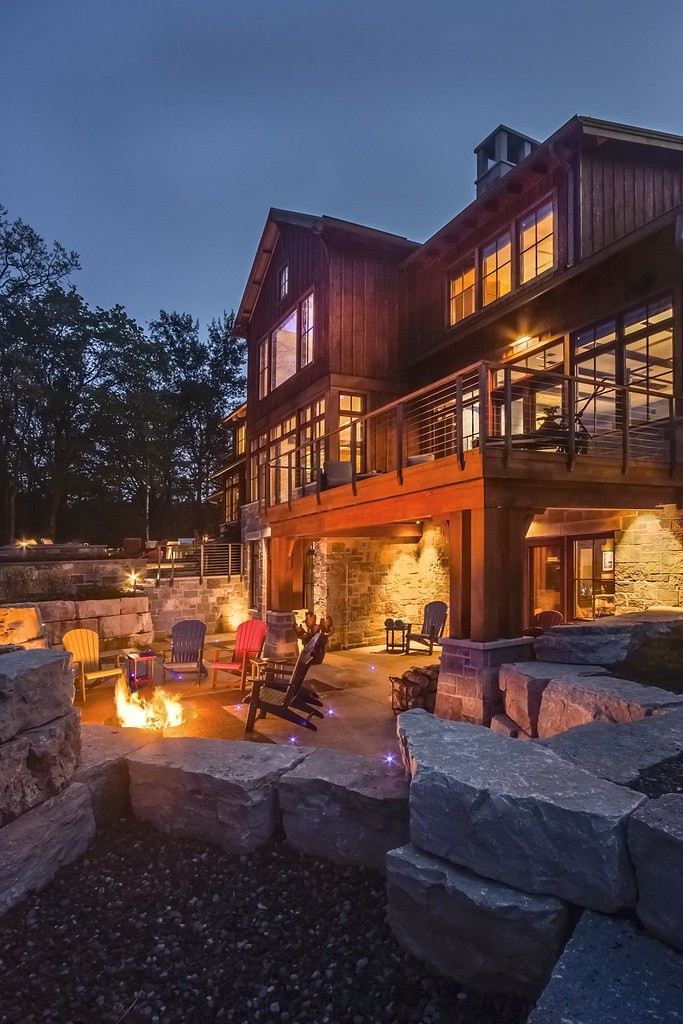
306,542,314,557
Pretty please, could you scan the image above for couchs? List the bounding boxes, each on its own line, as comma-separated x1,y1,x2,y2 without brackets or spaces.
323,460,354,490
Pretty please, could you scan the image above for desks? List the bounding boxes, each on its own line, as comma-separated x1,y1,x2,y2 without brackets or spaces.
384,627,410,651
249,657,287,680
123,646,156,689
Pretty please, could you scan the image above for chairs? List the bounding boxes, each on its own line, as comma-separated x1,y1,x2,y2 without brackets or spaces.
62,629,126,703
161,620,206,688
124,537,141,557
405,599,448,655
244,630,325,734
210,620,268,690
536,609,564,629
602,550,614,572
